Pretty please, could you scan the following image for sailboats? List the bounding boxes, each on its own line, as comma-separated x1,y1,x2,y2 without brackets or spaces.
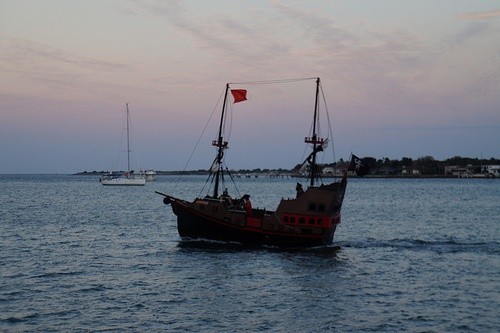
100,102,157,185
154,76,350,251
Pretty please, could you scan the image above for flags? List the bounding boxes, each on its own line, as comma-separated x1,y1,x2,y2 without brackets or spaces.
231,89,247,103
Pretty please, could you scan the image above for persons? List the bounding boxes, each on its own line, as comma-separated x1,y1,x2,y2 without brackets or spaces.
222,188,230,200
245,198,251,212
296,182,304,198
